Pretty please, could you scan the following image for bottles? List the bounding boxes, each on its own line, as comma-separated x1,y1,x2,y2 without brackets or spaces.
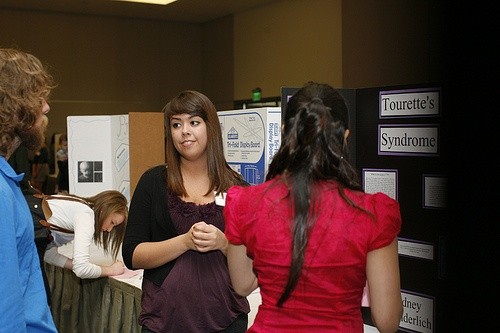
251,89,261,101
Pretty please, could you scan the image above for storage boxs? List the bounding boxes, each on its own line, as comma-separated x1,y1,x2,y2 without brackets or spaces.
66,105,283,205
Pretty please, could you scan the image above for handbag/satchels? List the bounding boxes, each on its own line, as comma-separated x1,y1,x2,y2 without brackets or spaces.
21,180,92,237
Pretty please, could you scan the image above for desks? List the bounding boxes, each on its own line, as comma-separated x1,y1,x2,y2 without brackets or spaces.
43,236,146,333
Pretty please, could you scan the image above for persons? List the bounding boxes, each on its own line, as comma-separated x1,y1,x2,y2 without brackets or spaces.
221,81,403,333
26,130,69,188
121,90,251,333
0,49,58,333
23,190,128,307
78,161,93,183
